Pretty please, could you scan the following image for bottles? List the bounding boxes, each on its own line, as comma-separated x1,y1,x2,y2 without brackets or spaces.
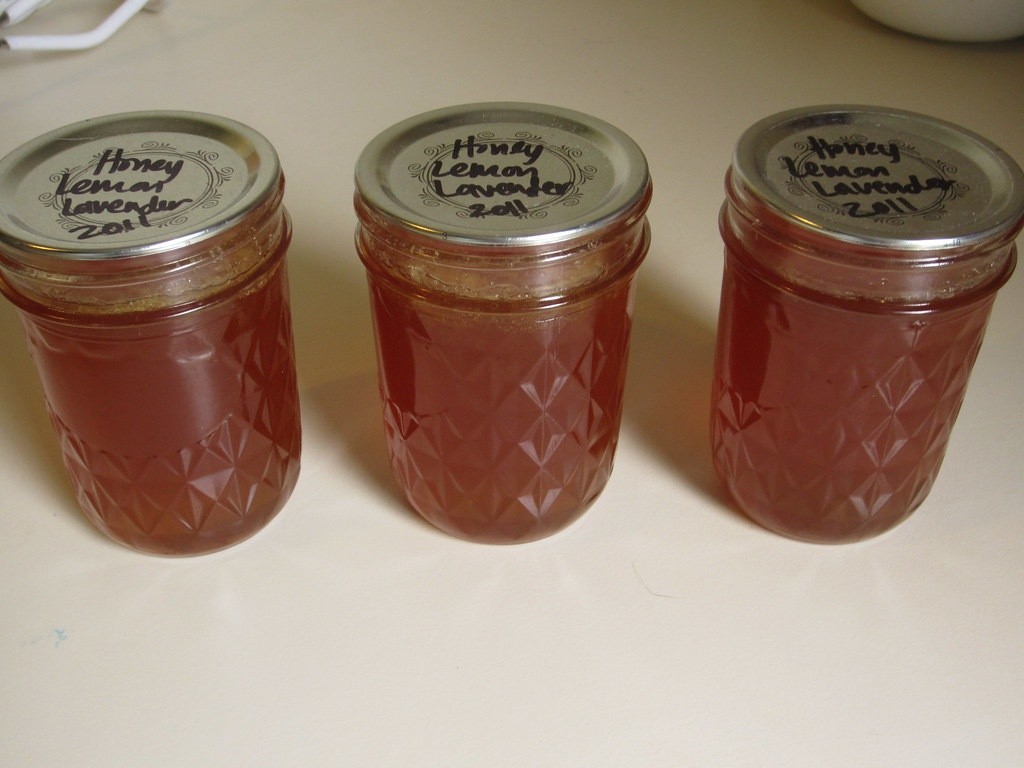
0,109,301,558
351,101,651,546
709,102,1024,545
